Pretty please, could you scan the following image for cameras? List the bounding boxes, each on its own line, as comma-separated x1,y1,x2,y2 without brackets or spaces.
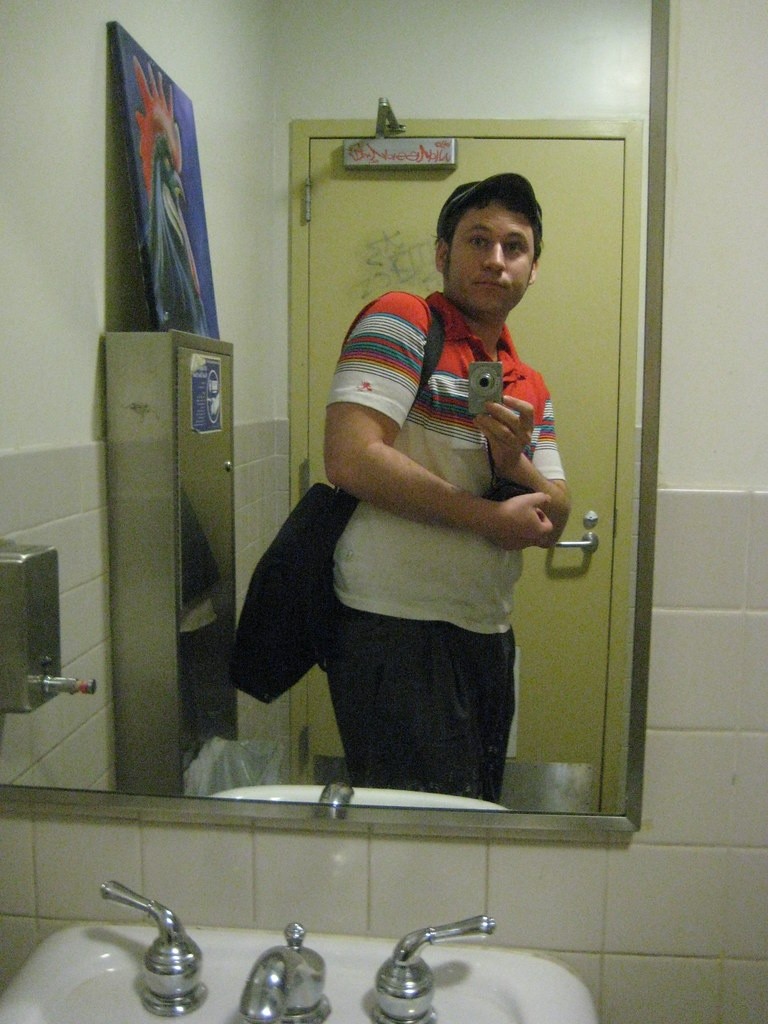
469,361,503,415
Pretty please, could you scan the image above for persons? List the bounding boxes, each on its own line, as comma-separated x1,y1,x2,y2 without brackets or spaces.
317,172,572,805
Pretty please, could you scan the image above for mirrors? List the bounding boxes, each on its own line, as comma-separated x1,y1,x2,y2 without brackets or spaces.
0,0,674,835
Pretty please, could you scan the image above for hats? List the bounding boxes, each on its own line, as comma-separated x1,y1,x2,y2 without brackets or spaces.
437,173,544,243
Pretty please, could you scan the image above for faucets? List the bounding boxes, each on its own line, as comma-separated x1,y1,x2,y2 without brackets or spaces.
320,784,352,817
242,923,331,1022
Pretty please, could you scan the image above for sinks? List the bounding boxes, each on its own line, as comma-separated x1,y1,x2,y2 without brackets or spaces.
206,784,510,811
1,925,596,1024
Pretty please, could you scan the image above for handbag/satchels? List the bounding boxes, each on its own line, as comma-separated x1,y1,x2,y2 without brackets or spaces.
228,482,360,704
